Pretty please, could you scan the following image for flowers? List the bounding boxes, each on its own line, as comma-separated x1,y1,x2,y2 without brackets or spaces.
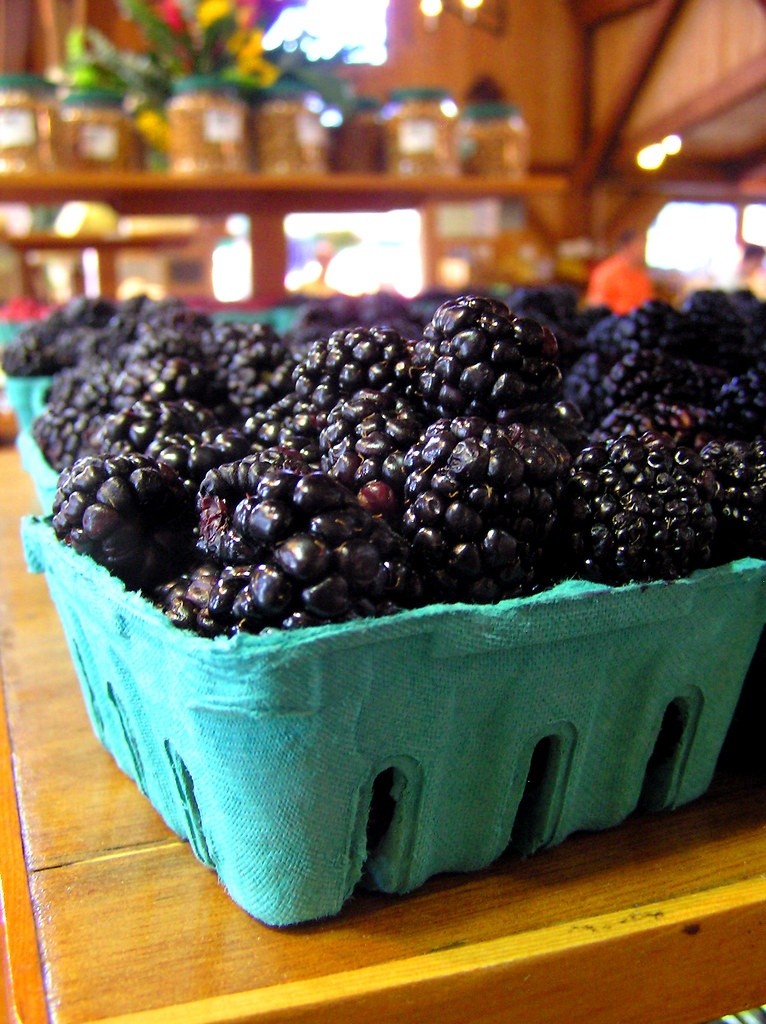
62,0,280,91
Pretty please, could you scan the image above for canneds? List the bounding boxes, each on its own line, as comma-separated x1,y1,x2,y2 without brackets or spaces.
0,74,530,184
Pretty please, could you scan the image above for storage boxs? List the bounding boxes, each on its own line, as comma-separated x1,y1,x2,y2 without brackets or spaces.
0,305,766,928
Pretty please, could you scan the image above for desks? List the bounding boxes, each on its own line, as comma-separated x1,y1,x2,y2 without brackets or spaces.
0,415,766,1024
